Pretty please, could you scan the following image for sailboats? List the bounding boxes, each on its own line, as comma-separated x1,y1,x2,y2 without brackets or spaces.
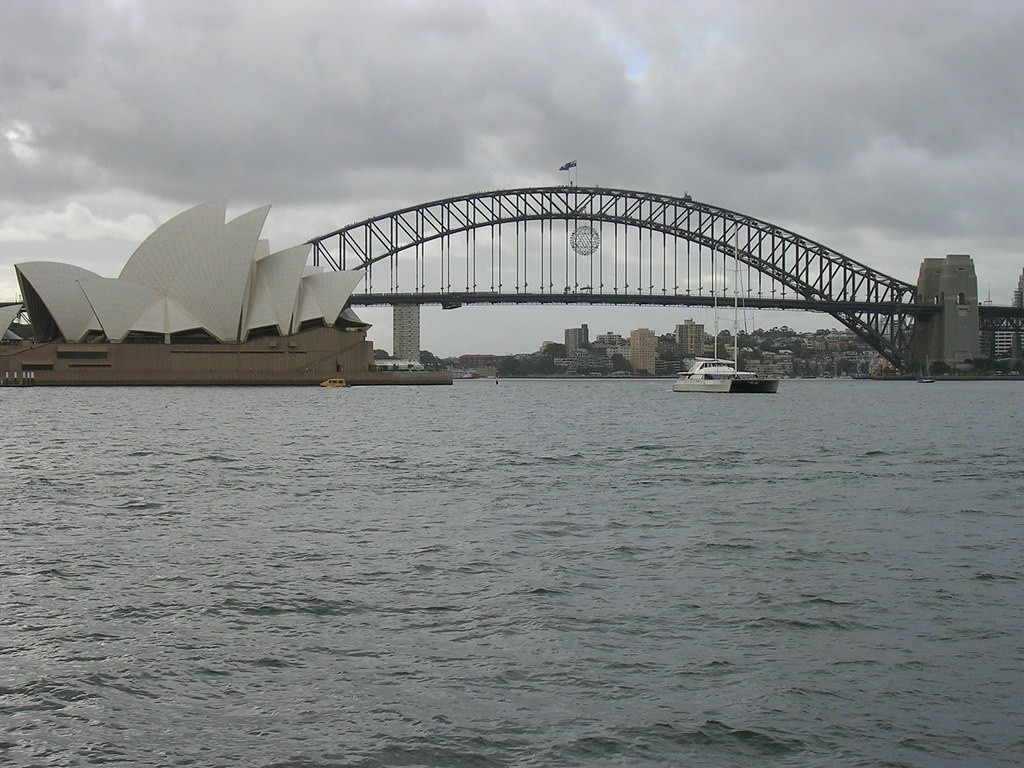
917,355,935,383
673,223,780,395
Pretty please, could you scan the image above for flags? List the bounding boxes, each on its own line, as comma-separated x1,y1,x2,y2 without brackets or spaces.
566,160,577,167
559,165,569,171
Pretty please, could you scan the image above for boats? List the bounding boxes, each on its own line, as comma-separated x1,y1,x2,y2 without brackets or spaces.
319,379,349,387
